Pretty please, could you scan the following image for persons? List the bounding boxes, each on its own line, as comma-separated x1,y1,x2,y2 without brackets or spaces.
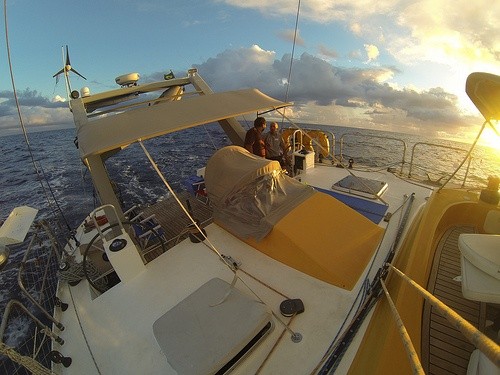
264,120,290,169
195,174,204,189
83,215,94,228
478,173,499,205
244,116,272,159
